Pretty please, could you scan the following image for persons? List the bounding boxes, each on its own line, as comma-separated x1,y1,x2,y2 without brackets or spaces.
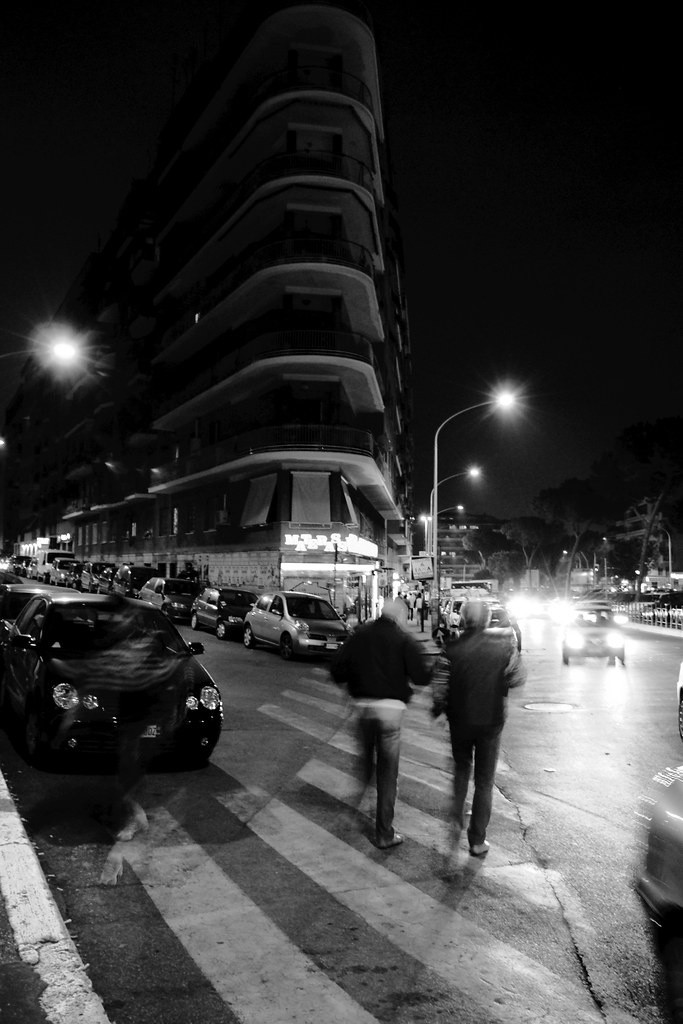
429,597,527,860
395,585,428,627
331,596,433,849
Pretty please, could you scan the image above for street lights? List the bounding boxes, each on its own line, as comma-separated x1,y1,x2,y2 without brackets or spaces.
433,396,511,636
430,469,481,588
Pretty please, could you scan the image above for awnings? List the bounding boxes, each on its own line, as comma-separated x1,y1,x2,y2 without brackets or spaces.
290,471,334,525
239,472,278,526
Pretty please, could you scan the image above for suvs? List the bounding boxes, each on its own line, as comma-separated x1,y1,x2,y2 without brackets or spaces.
65,563,82,589
644,592,683,614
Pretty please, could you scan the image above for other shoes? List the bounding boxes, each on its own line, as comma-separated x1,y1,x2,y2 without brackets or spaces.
378,833,405,848
470,839,490,855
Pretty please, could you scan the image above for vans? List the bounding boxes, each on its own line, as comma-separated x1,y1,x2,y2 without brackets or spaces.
82,561,115,593
36,549,75,584
114,565,160,599
50,557,79,586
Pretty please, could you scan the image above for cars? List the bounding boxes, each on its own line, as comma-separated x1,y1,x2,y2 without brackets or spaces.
0,584,83,675
191,586,260,640
0,553,37,579
242,590,356,660
0,592,222,771
139,577,201,621
572,602,616,620
583,590,661,605
0,570,26,588
97,568,117,594
440,590,521,652
562,606,626,666
